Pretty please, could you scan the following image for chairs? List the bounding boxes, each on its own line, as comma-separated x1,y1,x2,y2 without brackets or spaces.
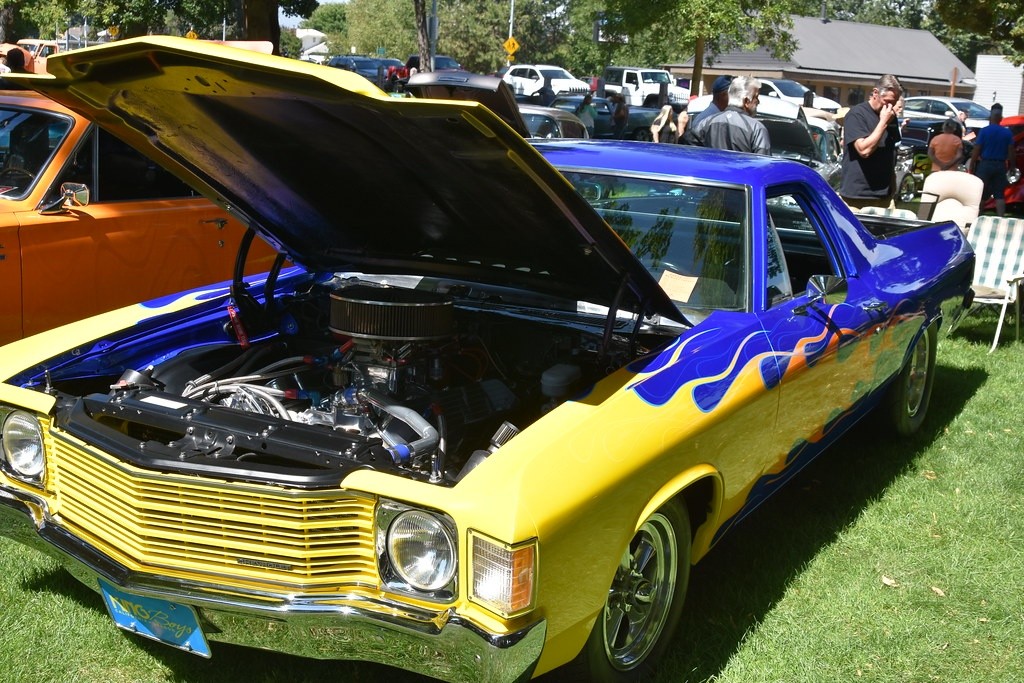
962,214,1024,358
915,167,985,245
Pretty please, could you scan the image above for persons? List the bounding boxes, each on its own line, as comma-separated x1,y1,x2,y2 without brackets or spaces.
0,48,35,74
831,74,909,210
543,85,555,107
604,94,629,140
575,94,598,139
650,105,675,143
928,102,1014,217
803,90,814,107
678,74,772,156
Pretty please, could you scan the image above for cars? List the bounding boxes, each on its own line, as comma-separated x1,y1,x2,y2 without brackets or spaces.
959,115,1024,218
755,77,841,115
375,57,404,79
358,63,929,175
902,95,992,159
0,40,293,336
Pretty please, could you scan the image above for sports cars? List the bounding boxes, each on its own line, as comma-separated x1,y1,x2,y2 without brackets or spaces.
0,33,979,682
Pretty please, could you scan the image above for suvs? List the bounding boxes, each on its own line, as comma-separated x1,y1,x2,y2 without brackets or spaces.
402,55,477,74
500,63,591,96
327,55,388,84
601,66,691,114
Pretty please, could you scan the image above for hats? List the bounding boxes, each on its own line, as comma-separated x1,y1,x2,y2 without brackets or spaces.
712,75,733,93
7,48,24,67
